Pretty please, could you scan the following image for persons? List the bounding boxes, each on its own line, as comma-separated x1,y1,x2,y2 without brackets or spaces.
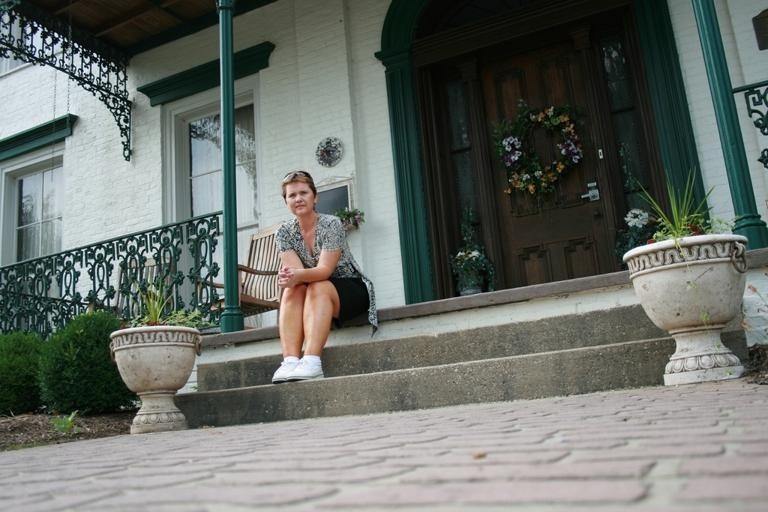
272,171,377,384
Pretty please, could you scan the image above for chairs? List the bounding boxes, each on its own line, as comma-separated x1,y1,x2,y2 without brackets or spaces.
86,256,177,321
197,224,283,330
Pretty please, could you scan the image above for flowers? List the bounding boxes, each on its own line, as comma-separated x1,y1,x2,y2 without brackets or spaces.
489,96,585,199
447,204,495,295
617,208,738,245
332,205,366,233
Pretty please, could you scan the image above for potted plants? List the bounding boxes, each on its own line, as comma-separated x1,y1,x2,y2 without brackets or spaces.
620,164,750,388
106,274,217,435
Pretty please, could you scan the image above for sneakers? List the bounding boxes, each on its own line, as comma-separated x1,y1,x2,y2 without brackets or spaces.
270,356,326,382
